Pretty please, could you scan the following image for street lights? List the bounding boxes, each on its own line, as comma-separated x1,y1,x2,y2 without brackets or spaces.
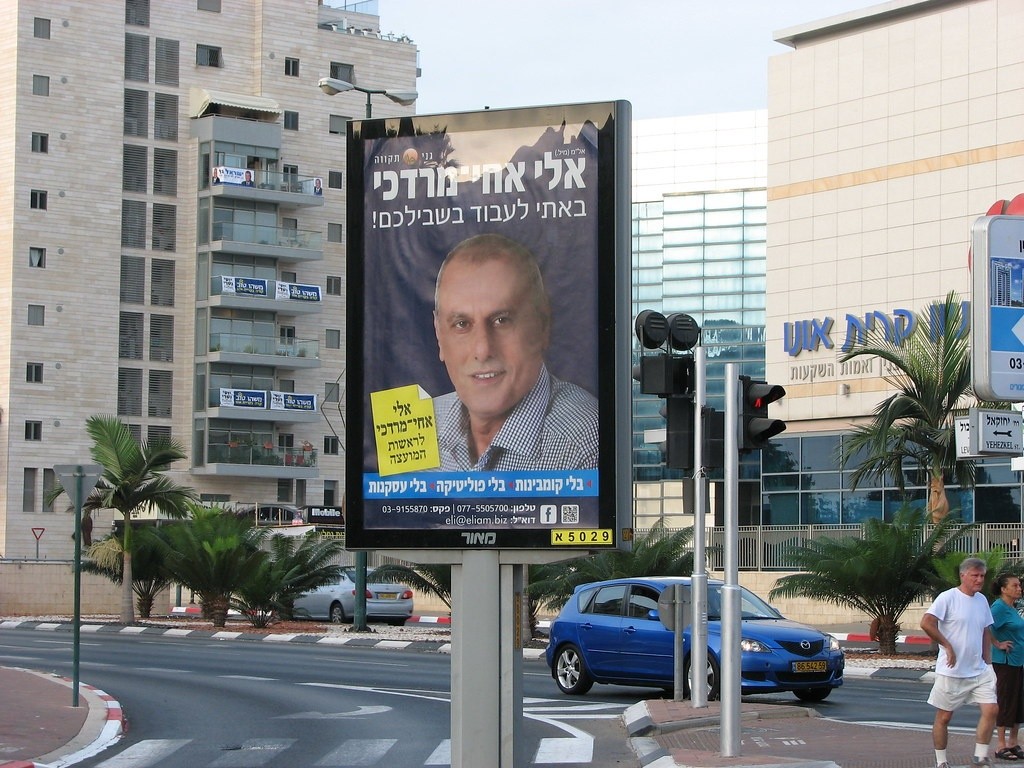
318,75,419,632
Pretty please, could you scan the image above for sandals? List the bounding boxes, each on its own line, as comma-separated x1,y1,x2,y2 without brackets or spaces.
995,745,1024,761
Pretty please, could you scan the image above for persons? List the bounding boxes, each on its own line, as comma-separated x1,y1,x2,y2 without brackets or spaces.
920,557,999,768
212,168,220,183
242,171,254,186
314,179,322,194
988,572,1024,760
427,234,598,472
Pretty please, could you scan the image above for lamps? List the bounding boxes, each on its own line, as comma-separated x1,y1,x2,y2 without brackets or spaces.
839,383,850,394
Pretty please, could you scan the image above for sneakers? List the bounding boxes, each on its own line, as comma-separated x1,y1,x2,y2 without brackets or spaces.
935,762,952,768
971,757,995,768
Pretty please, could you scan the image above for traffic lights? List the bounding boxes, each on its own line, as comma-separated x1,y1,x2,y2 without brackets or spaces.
742,378,787,449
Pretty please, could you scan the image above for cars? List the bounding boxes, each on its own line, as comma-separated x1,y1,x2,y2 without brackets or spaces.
293,564,414,626
545,576,846,704
